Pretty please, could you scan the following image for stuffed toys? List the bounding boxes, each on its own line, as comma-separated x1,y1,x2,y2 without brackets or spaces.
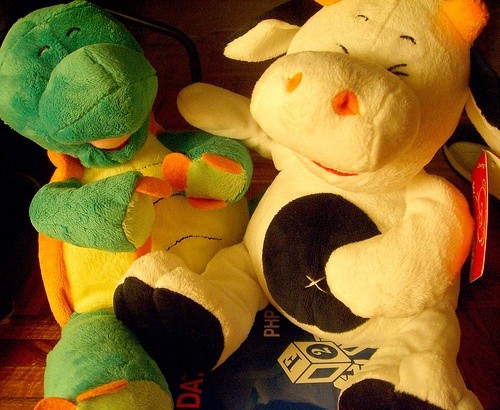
0,0,252,410
111,0,490,410
